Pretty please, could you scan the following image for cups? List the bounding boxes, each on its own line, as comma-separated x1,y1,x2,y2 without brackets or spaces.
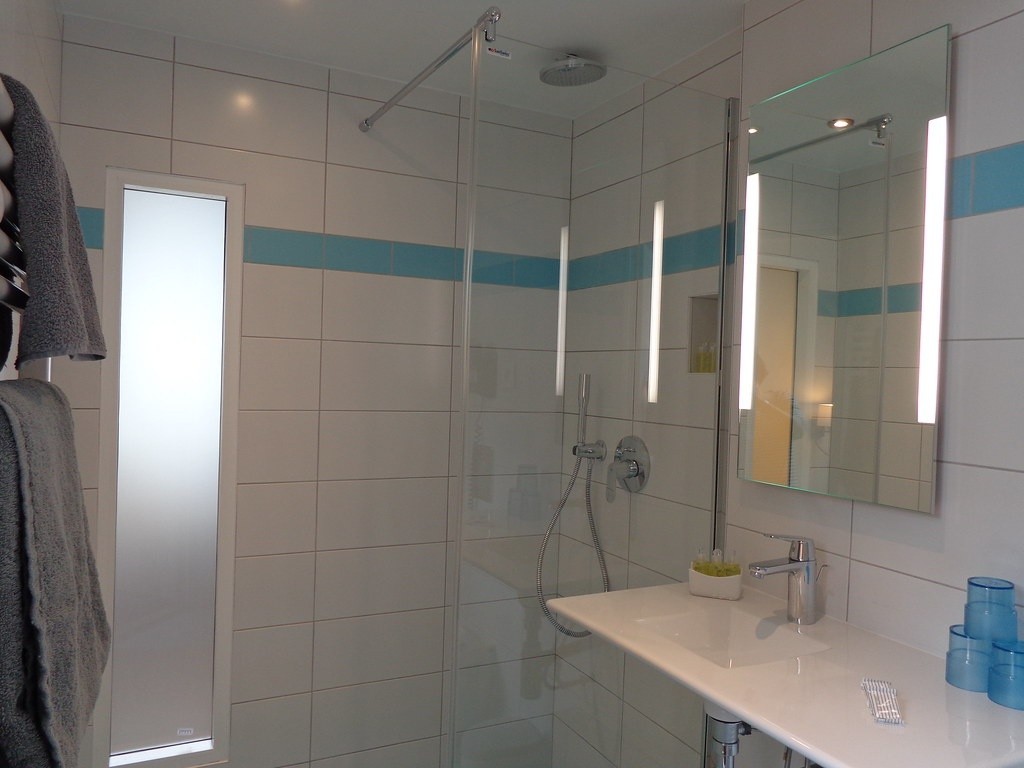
963,576,1018,642
987,640,1024,711
945,624,989,693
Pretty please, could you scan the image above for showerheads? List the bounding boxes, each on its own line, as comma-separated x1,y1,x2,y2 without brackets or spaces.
539,54,608,88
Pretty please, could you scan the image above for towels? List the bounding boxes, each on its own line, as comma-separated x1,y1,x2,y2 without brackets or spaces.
1,376,108,768
0,74,110,361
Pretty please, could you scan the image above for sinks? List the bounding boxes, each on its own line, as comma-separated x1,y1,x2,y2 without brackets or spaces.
636,606,832,673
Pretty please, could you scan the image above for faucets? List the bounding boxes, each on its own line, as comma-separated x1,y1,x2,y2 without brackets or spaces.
747,535,818,624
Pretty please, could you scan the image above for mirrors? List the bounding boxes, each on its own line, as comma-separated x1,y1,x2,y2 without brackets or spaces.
736,23,953,517
